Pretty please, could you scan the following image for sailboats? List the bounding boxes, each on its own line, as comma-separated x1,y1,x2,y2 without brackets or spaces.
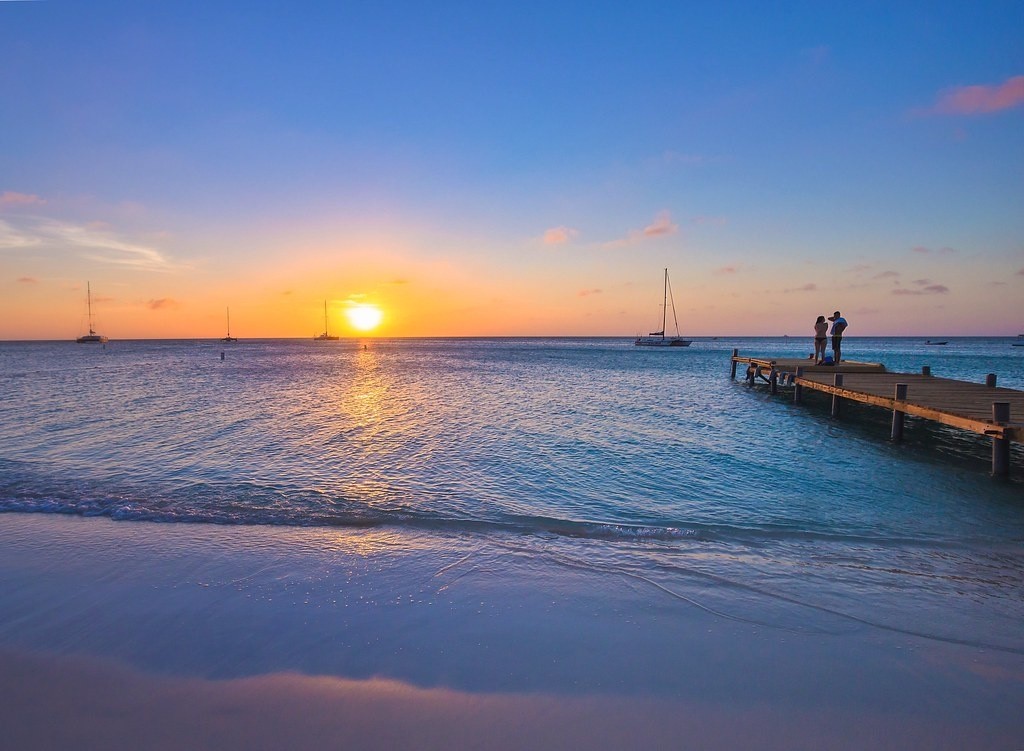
79,279,111,345
313,301,339,343
633,267,696,348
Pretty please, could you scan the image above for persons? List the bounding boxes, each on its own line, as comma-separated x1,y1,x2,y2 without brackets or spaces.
813,315,828,365
828,311,848,363
